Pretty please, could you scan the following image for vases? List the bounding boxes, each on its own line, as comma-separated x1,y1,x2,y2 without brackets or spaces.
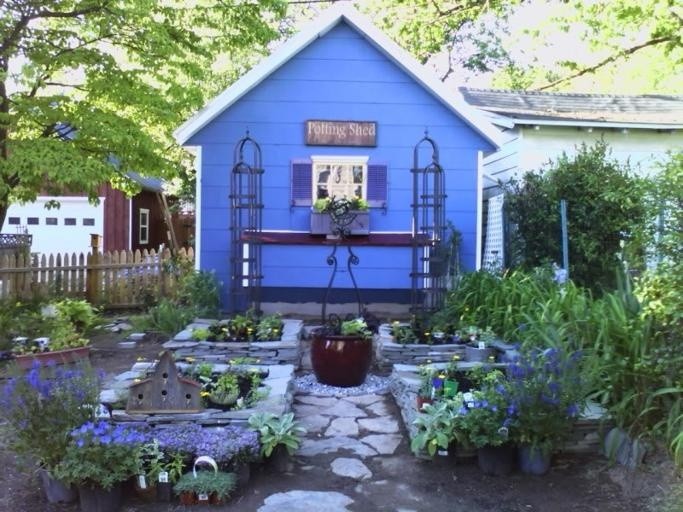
234,463,252,486
36,467,76,503
474,439,552,476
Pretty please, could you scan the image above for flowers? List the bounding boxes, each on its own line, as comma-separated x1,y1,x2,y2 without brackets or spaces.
194,424,260,467
457,322,589,460
0,355,106,467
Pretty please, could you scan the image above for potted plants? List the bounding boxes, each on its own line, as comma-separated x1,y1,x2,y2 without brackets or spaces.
308,318,374,388
77,442,240,511
310,195,371,236
409,397,469,470
248,409,308,472
12,320,94,370
